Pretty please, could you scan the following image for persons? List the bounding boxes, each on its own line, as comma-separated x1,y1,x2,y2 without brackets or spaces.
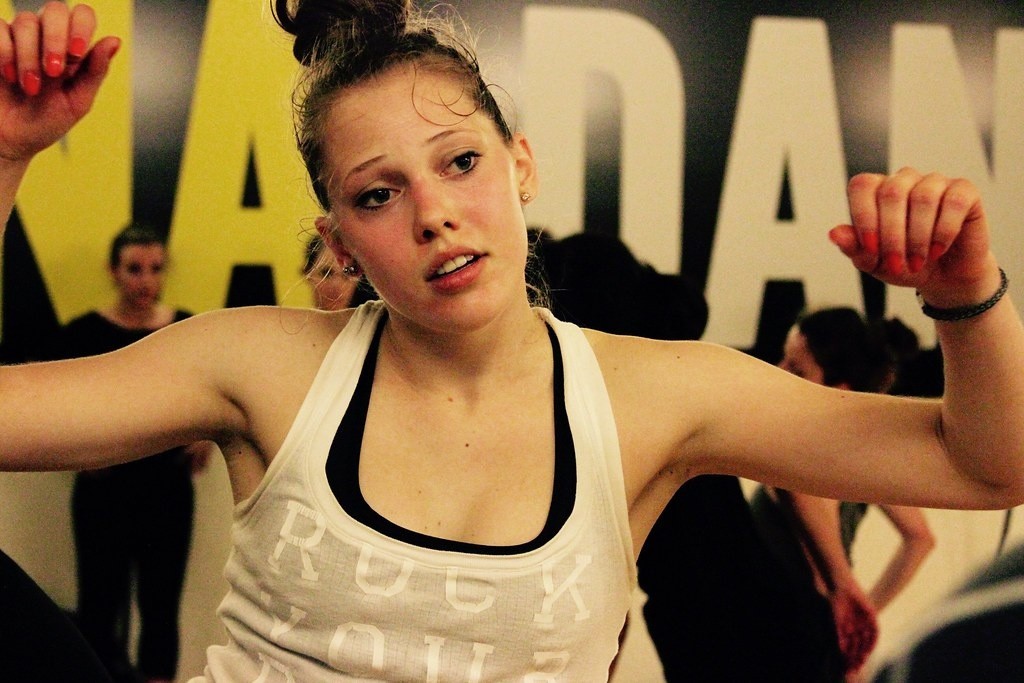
298,234,361,311
3,2,1023,683
528,225,880,682
17,222,218,683
746,304,938,642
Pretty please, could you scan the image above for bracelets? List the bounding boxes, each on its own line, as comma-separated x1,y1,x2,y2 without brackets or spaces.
913,267,1007,321
